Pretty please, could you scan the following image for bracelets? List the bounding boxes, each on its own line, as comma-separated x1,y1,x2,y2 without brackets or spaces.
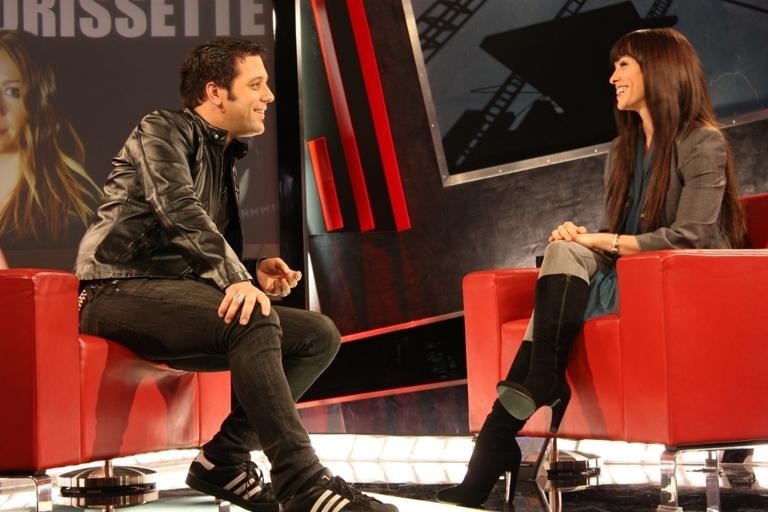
611,232,622,258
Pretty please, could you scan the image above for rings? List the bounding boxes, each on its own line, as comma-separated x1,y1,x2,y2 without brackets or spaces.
232,294,245,304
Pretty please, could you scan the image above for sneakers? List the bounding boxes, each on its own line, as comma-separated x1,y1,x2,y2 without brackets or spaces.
280,467,399,512
186,449,279,512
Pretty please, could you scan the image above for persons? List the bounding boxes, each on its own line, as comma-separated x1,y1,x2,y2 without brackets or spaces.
434,27,756,506
0,28,104,271
64,33,401,512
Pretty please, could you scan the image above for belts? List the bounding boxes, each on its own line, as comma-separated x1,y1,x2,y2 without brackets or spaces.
78,279,111,317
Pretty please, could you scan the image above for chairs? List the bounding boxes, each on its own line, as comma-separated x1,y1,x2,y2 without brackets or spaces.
0,248,234,512
462,192,768,512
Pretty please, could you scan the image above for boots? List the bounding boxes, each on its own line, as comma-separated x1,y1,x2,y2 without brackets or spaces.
436,340,532,505
496,273,591,433
